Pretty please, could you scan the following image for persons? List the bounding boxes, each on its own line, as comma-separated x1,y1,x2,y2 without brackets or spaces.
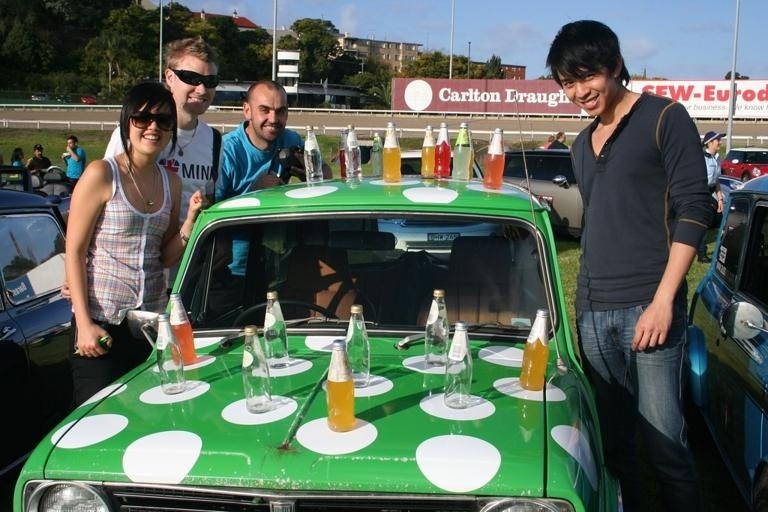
60,37,224,303
548,132,568,150
61,135,86,194
543,136,555,149
11,148,33,181
214,79,333,203
696,132,727,263
64,81,210,407
28,144,51,179
545,20,714,512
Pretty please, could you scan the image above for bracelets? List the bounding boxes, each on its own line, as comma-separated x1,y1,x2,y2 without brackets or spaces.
718,199,724,201
176,230,189,243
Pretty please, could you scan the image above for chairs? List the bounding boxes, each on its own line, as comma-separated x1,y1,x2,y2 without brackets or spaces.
416,237,534,328
278,244,374,323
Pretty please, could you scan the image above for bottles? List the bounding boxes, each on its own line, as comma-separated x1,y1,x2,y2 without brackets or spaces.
304,121,506,190
243,325,273,413
326,340,356,432
264,291,289,369
424,290,449,367
444,321,473,408
156,313,185,394
346,305,371,388
170,293,197,365
520,309,550,391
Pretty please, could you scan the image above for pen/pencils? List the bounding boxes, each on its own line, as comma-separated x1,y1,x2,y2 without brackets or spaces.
72,336,110,354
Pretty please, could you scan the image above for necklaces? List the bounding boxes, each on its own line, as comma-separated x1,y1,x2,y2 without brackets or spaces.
124,152,159,215
171,120,197,156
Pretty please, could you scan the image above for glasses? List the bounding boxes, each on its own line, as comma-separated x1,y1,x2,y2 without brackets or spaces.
168,67,220,89
132,111,176,131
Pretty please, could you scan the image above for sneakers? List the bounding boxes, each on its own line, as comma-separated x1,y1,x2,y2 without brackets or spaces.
698,254,711,263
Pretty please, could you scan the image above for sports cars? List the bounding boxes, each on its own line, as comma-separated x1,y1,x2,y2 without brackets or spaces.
0,162,80,224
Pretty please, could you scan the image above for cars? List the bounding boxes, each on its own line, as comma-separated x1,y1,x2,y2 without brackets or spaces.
0,188,71,512
501,149,584,243
374,148,506,267
12,94,620,512
686,146,768,511
31,91,98,104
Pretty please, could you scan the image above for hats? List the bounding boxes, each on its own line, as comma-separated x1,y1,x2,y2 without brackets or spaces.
703,131,726,145
34,144,43,150
